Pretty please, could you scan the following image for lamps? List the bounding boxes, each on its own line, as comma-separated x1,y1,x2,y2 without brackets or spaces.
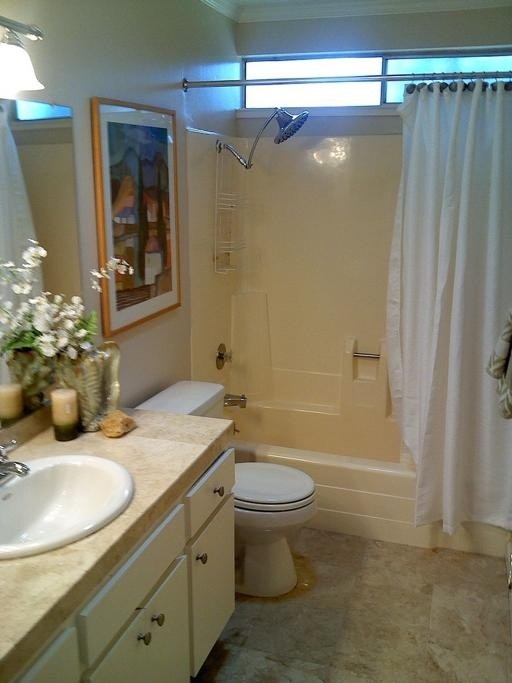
0,13,47,100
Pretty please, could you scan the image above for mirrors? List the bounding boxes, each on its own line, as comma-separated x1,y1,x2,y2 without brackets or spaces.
0,95,83,431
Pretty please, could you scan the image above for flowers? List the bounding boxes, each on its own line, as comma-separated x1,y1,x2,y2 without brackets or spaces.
0,237,135,397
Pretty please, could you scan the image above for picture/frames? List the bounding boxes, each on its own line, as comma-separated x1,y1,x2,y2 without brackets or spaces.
90,96,182,339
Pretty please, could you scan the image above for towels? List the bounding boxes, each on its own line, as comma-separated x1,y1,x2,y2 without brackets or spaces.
486,315,512,419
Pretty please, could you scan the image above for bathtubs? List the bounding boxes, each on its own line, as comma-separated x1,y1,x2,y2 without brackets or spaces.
229,399,440,550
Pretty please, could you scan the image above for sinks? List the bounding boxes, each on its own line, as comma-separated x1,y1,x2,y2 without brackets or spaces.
0,456,133,560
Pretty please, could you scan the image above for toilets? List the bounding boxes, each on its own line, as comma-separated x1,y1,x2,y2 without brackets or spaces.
133,379,318,597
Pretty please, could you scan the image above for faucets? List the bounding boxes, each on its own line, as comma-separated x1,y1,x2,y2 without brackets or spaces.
224,393,247,409
0,439,31,484
